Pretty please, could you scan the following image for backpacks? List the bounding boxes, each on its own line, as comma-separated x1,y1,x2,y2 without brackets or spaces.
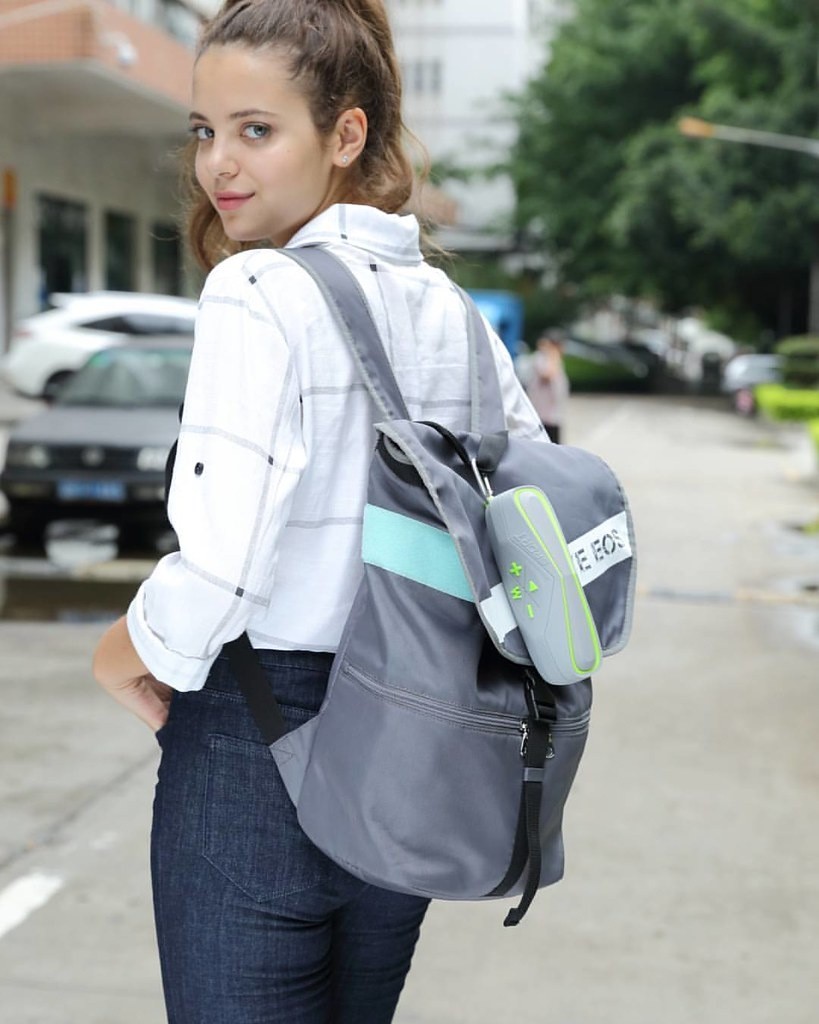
161,238,634,930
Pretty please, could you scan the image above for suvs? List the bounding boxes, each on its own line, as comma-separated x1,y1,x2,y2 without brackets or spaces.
4,285,200,407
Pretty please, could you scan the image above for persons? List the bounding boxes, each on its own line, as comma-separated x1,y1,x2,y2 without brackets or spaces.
95,0,552,1024
499,316,569,445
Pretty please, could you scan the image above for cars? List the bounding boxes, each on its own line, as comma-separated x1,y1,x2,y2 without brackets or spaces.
0,335,200,548
